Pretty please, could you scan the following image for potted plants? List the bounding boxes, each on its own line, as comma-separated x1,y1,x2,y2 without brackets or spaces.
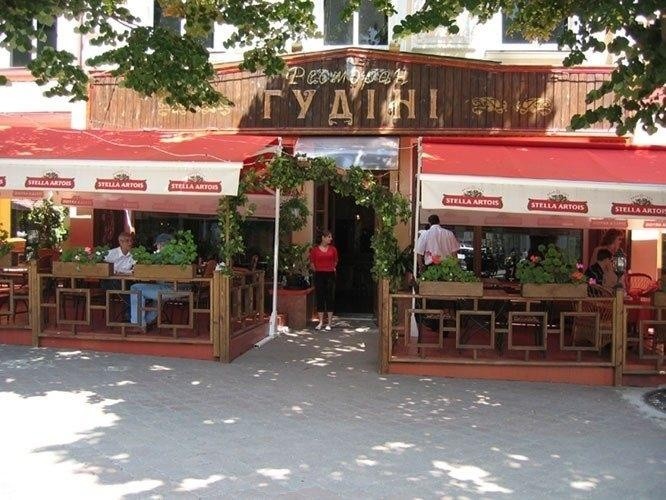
265,240,319,330
130,228,197,280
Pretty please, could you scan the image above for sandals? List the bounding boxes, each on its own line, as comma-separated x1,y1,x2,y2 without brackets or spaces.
315,325,331,331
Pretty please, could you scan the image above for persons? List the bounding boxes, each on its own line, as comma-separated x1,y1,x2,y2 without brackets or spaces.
100,231,194,333
414,215,459,337
584,228,624,297
308,229,340,330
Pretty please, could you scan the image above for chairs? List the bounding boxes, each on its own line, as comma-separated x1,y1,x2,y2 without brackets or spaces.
624,273,655,324
567,279,615,357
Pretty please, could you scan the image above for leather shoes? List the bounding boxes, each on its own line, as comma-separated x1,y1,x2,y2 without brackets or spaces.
126,326,143,334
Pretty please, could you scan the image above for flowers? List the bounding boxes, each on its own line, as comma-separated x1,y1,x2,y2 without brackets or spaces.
417,252,484,282
513,243,597,285
60,245,109,266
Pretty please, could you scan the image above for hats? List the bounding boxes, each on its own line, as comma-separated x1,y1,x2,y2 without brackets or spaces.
154,233,172,246
